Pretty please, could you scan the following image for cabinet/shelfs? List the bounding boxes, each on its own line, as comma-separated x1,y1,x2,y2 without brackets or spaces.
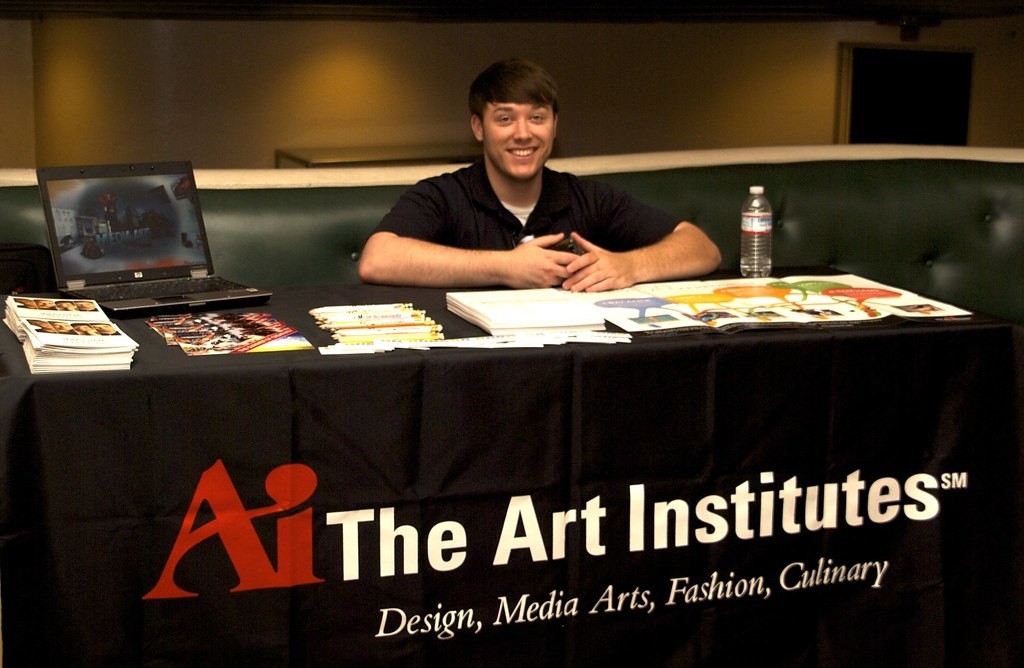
2,265,1024,666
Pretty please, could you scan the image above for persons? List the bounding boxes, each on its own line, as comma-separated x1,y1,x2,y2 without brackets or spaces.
356,54,724,294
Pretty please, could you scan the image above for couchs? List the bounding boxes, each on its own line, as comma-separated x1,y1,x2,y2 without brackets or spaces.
0,139,1024,325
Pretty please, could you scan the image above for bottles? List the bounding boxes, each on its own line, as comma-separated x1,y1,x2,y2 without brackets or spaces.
739,185,772,277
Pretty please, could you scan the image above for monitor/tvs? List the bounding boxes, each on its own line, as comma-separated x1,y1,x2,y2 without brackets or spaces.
840,39,979,148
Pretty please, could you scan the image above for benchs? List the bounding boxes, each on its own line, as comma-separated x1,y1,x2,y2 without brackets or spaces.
273,141,482,166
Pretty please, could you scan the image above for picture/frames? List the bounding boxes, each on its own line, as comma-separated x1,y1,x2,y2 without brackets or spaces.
838,41,976,144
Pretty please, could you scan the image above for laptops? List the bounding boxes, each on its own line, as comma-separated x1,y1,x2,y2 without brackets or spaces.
36,160,274,316
